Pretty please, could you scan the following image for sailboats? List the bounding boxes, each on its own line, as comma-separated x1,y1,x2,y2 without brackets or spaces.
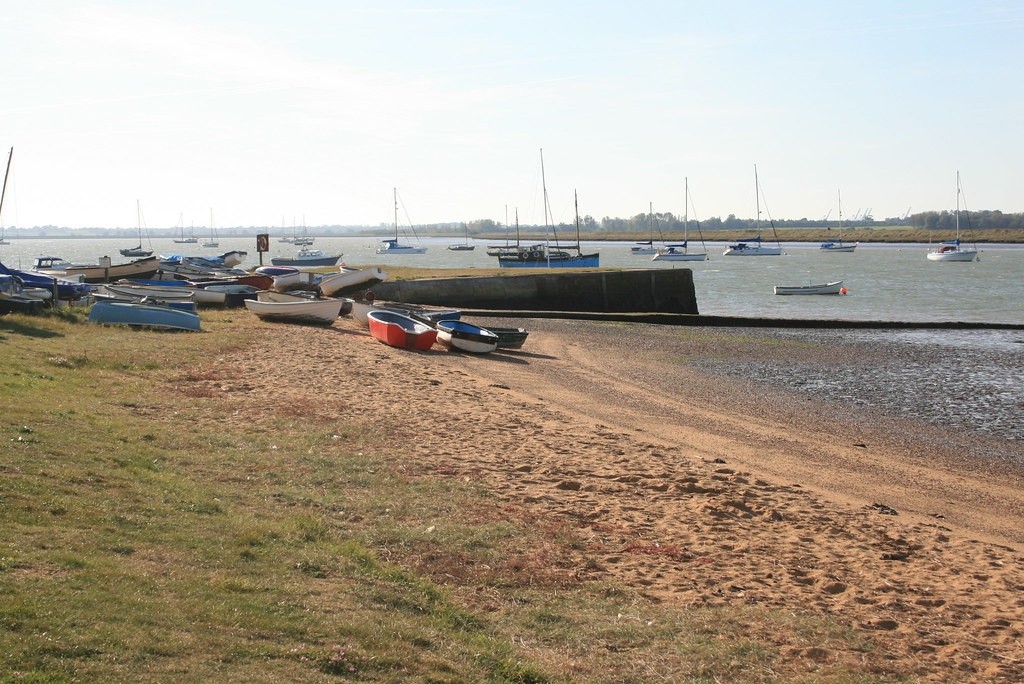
485,204,534,257
172,211,198,243
631,201,671,257
376,186,428,253
201,207,220,247
499,146,603,268
721,163,787,257
444,223,476,251
647,175,712,262
927,170,982,263
817,189,859,253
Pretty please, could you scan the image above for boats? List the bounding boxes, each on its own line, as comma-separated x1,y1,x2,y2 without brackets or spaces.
85,299,201,332
244,297,345,326
378,306,462,323
255,289,354,316
0,148,346,310
437,319,500,355
267,244,345,270
409,312,529,351
773,279,847,296
316,267,388,299
365,310,438,352
